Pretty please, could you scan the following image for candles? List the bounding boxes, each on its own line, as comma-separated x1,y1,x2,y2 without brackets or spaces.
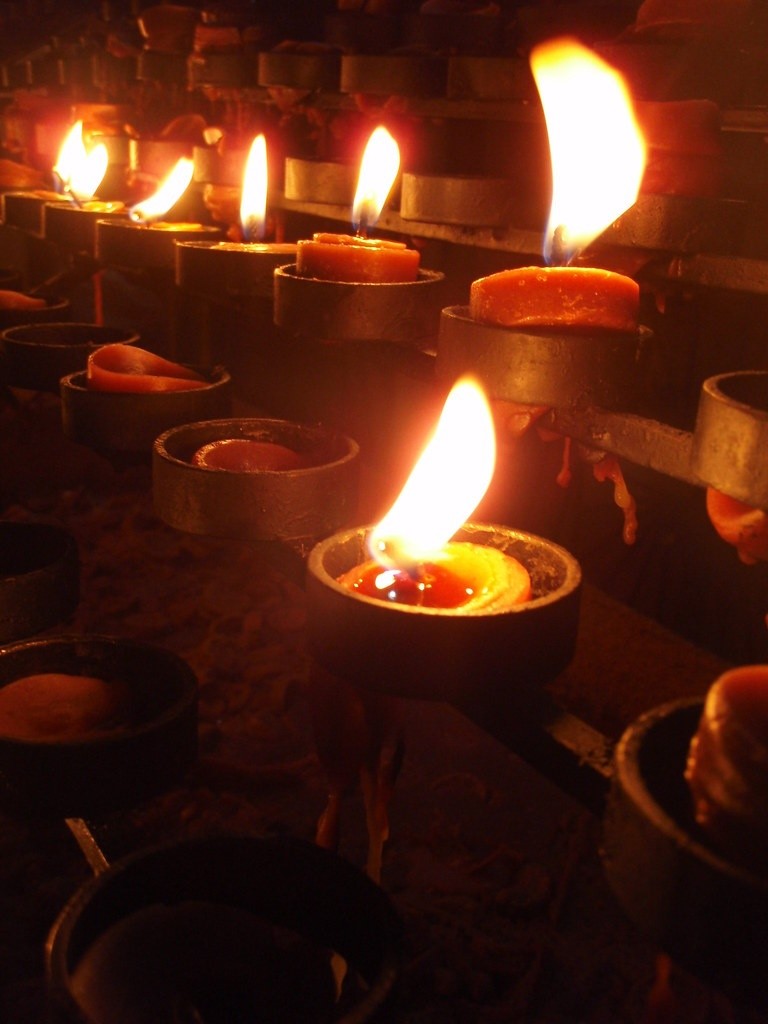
0,672,137,741
335,369,531,614
192,437,307,471
635,0,753,46
193,21,267,54
71,100,135,136
685,662,768,866
128,154,203,231
209,134,297,254
296,124,420,283
470,38,650,334
32,119,87,199
63,143,126,212
86,344,210,393
137,4,197,54
637,97,726,200
